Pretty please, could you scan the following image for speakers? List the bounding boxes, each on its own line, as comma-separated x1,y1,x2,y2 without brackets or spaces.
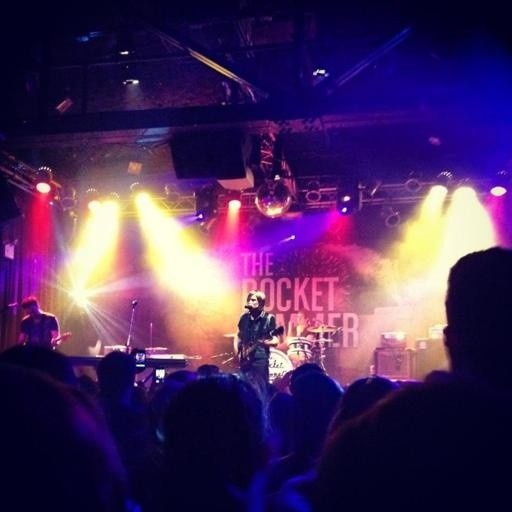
415,341,448,381
374,350,414,380
169,125,251,179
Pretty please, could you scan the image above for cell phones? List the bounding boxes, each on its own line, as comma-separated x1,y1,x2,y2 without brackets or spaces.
135,349,146,369
155,368,165,384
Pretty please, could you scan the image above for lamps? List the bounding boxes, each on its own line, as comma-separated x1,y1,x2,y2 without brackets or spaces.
377,192,399,227
228,191,242,211
404,165,508,198
305,174,322,202
59,180,156,212
34,154,56,195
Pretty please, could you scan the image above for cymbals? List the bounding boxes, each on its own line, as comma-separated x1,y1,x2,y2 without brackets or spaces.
308,326,338,332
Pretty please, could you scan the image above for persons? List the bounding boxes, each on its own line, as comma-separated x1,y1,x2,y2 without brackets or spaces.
17,295,73,351
311,243,510,511
233,289,279,383
1,344,454,511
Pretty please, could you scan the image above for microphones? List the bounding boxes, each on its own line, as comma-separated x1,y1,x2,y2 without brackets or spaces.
245,305,252,309
132,299,138,306
8,302,18,308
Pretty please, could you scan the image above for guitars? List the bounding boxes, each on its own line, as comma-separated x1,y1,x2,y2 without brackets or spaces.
234,326,284,364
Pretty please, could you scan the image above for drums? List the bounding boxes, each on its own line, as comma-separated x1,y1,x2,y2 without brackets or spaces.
268,350,292,385
288,339,313,360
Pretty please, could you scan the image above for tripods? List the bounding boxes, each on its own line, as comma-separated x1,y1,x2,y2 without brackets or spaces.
309,333,329,375
